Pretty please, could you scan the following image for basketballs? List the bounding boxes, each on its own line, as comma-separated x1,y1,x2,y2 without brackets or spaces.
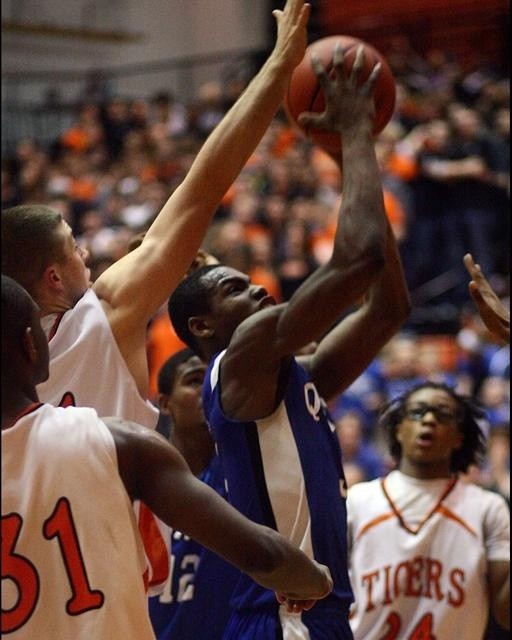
283,34,397,149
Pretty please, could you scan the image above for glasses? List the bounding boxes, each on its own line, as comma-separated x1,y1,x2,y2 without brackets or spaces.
399,399,458,425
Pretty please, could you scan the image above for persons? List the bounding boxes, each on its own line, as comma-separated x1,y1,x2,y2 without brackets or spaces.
1,0,309,591
165,43,414,640
131,247,221,639
460,252,510,344
278,381,512,640
0,272,333,639
